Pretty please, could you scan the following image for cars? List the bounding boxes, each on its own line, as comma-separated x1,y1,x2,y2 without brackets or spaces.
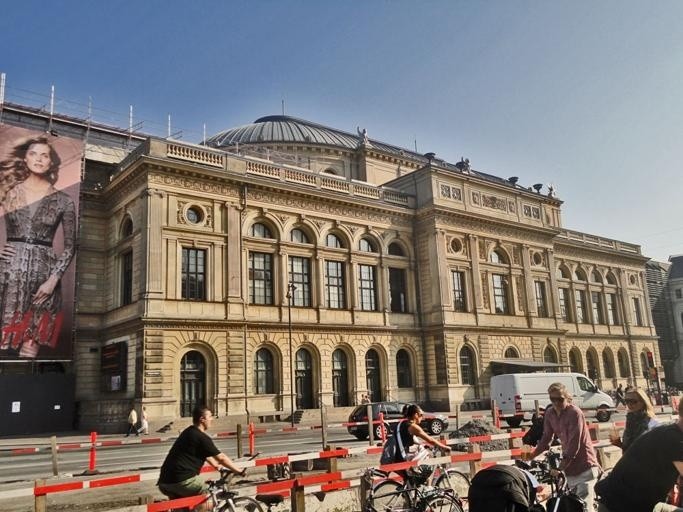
348,400,449,441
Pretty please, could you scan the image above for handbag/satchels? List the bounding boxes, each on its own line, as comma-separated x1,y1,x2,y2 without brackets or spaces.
545,471,588,512
405,446,435,486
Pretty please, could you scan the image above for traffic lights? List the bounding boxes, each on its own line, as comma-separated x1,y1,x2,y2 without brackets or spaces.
647,351,654,368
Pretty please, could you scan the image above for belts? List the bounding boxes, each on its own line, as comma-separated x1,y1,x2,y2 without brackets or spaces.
7,237,52,246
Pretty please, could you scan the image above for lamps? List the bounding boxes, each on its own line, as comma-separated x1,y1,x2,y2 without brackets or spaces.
50,129,58,137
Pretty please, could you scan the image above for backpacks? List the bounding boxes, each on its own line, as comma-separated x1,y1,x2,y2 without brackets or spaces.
380,424,410,464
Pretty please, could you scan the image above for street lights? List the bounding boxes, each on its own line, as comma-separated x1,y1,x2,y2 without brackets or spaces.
286,282,297,428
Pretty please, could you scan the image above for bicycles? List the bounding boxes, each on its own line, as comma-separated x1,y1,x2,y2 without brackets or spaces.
154,466,284,512
361,445,613,512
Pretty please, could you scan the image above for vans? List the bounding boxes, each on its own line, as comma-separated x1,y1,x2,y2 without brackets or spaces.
489,372,616,428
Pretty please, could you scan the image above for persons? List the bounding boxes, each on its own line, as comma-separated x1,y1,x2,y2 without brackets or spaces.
389,403,452,501
137,406,149,434
156,407,247,512
361,393,365,405
124,407,139,437
0,134,77,358
468,381,682,512
364,395,371,403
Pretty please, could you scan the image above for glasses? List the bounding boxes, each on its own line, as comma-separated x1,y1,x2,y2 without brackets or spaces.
551,398,566,402
625,399,641,404
